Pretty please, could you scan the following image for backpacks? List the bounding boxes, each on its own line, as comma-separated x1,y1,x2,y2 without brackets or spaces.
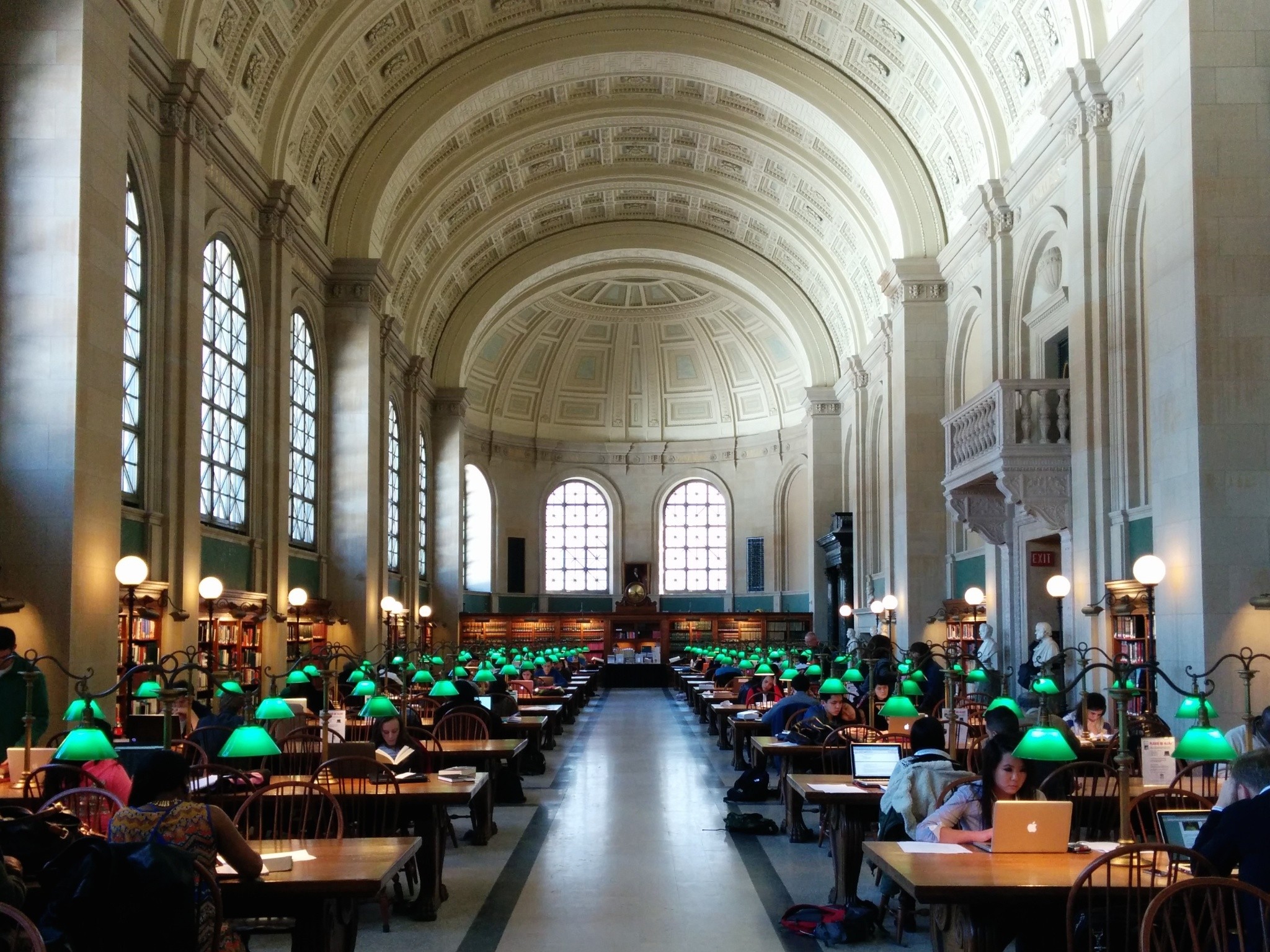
781,900,879,947
727,769,770,802
723,812,779,836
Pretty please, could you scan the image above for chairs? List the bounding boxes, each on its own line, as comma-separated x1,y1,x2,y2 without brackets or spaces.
712,674,1270,952
0,670,546,952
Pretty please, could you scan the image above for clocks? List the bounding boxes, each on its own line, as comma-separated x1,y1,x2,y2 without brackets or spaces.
625,581,647,603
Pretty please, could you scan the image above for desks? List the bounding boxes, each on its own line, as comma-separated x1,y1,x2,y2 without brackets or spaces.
669,665,1115,844
0,772,489,920
597,663,669,689
787,774,1227,907
173,669,606,846
863,842,1238,952
216,835,423,952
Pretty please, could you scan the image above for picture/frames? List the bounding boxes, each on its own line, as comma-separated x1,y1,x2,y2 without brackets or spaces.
622,561,651,596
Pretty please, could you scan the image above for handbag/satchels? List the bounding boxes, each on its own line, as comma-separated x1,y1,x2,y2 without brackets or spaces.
520,744,546,775
191,769,271,795
774,716,859,746
495,767,527,803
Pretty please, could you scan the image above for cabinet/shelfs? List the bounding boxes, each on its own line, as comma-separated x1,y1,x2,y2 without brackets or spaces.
197,589,268,705
605,613,668,661
943,596,986,671
1105,578,1157,715
115,580,170,725
286,596,330,670
668,610,813,658
458,611,605,658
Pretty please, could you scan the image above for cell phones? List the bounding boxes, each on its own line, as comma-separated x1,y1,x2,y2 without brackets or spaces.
1143,869,1168,876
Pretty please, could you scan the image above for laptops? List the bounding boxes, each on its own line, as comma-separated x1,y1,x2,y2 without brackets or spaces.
511,680,533,695
539,676,554,686
7,747,58,784
128,714,181,747
850,743,904,785
507,690,517,703
1157,809,1240,877
972,801,1073,853
474,695,493,711
732,676,753,695
328,743,378,779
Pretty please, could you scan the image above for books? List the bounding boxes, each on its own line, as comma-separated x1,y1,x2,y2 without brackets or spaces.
670,619,807,657
737,709,759,719
438,766,476,776
1112,613,1157,719
374,745,414,766
670,656,681,663
215,854,269,876
116,614,314,734
614,628,660,639
392,618,604,647
947,623,980,699
439,774,476,782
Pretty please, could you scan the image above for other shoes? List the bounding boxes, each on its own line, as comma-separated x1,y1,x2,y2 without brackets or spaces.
748,758,751,762
893,915,916,932
410,902,437,921
575,708,580,715
819,814,830,825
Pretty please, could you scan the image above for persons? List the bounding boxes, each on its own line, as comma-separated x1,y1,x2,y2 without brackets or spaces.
0,626,49,775
1032,622,1061,677
869,622,888,636
44,652,588,951
672,633,1270,951
978,623,998,669
846,628,861,668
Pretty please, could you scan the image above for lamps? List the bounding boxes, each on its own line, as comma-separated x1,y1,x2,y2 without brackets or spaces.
1175,646,1270,752
1011,654,1239,868
0,557,590,789
683,554,1167,759
